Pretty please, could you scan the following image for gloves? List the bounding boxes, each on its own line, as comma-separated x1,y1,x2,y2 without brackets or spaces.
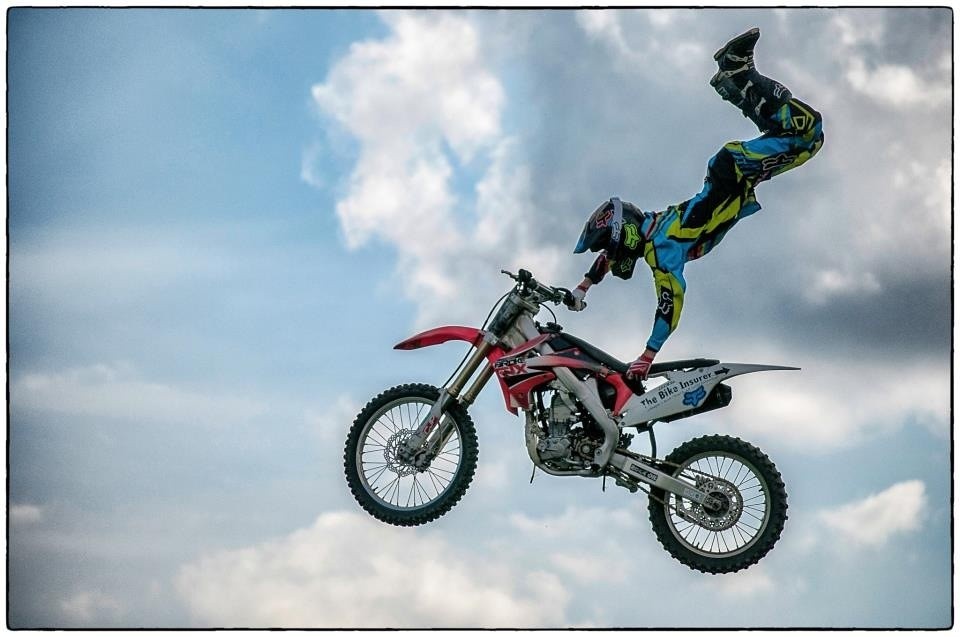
568,276,592,312
626,349,655,381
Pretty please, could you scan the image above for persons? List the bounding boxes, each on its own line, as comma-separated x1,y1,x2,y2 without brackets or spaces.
566,26,824,381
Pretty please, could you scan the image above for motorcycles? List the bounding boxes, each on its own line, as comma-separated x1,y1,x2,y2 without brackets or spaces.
342,267,804,576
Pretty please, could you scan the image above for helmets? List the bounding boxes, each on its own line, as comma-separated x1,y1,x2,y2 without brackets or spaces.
573,197,644,260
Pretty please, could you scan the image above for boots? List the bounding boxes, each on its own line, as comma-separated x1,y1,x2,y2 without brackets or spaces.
714,27,791,119
710,70,769,131
